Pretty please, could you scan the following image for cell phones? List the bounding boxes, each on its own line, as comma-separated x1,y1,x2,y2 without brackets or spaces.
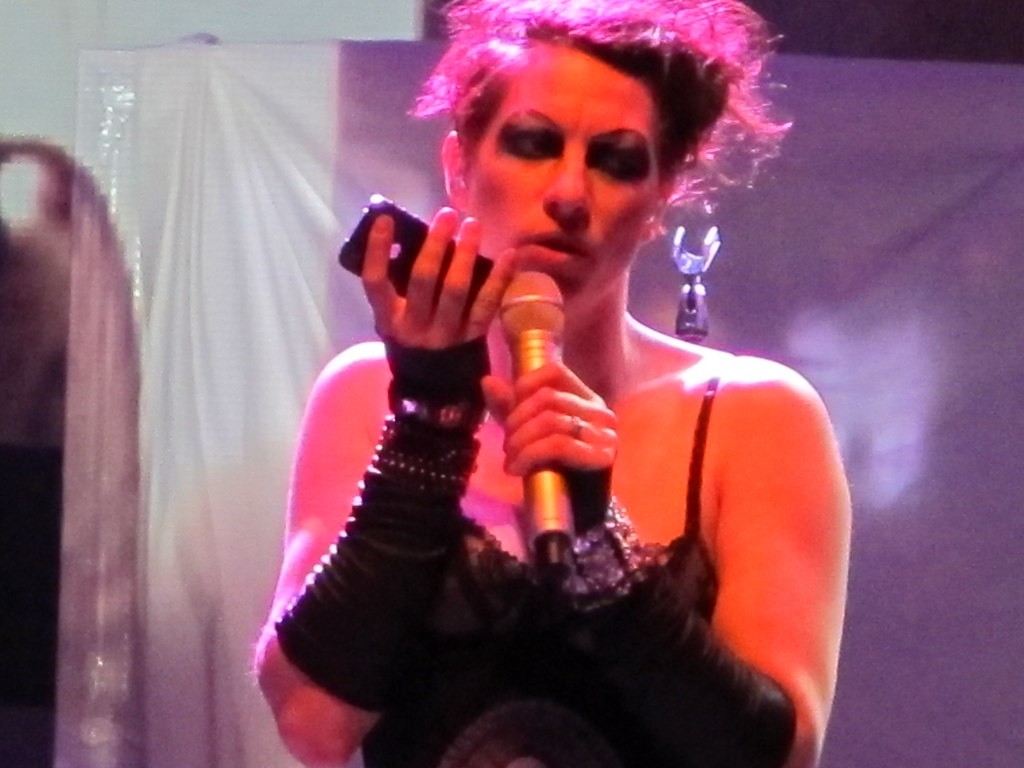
337,193,494,316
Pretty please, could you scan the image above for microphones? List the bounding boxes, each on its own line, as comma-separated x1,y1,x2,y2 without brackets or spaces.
498,273,573,568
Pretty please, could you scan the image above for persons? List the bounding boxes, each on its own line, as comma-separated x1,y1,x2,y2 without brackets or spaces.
254,0,853,768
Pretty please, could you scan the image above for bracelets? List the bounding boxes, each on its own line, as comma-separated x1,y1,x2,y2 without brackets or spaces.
562,495,669,612
